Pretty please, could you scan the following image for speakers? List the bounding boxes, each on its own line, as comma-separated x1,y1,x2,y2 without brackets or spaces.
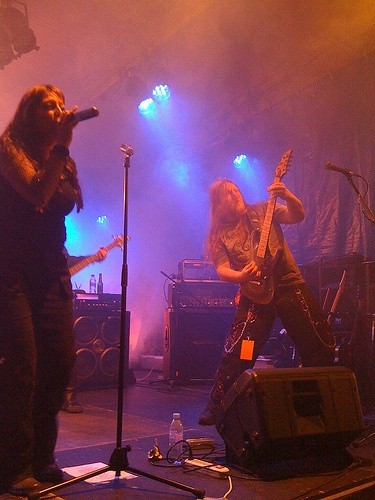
165,309,237,380
318,261,375,409
214,366,365,479
71,309,130,392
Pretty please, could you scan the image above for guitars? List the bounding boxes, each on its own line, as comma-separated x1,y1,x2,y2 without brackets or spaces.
240,148,296,303
69,233,132,275
327,270,348,325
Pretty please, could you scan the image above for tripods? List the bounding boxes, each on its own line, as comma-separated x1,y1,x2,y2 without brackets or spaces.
26,144,204,500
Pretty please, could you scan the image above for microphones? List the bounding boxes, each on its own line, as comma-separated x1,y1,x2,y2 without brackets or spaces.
324,162,353,176
69,106,100,120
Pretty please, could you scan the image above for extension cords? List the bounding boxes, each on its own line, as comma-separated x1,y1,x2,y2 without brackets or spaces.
183,457,230,479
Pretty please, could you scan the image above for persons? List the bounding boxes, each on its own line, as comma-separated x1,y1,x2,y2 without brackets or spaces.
197,177,341,426
61,245,108,413
0,82,83,495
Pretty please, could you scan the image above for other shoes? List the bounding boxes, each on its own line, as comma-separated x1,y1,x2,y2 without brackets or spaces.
199,408,214,425
5,475,42,497
34,459,64,483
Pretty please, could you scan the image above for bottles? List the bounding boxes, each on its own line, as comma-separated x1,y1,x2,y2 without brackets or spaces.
97,273,103,294
90,274,96,294
168,412,184,464
